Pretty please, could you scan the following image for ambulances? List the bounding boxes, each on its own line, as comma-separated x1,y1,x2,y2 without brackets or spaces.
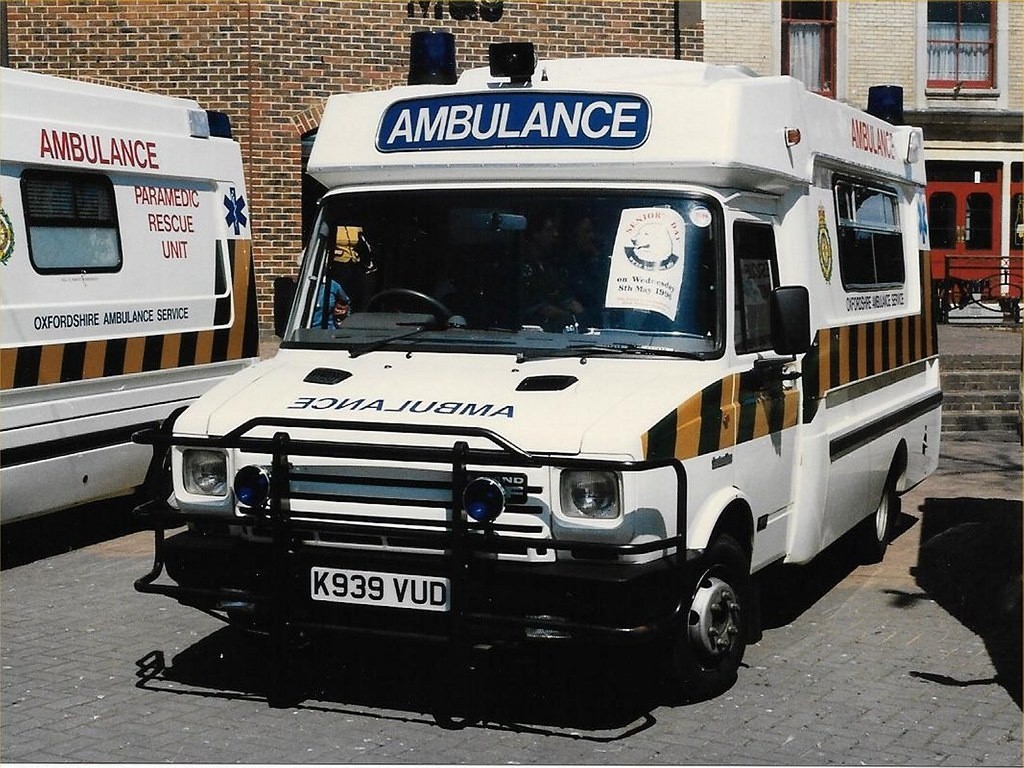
0,68,258,531
130,33,942,684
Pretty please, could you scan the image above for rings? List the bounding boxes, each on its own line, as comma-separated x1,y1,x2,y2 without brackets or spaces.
340,310,341,313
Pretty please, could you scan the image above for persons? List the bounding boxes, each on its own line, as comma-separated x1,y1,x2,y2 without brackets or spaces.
294,244,353,329
513,202,599,329
568,243,661,333
